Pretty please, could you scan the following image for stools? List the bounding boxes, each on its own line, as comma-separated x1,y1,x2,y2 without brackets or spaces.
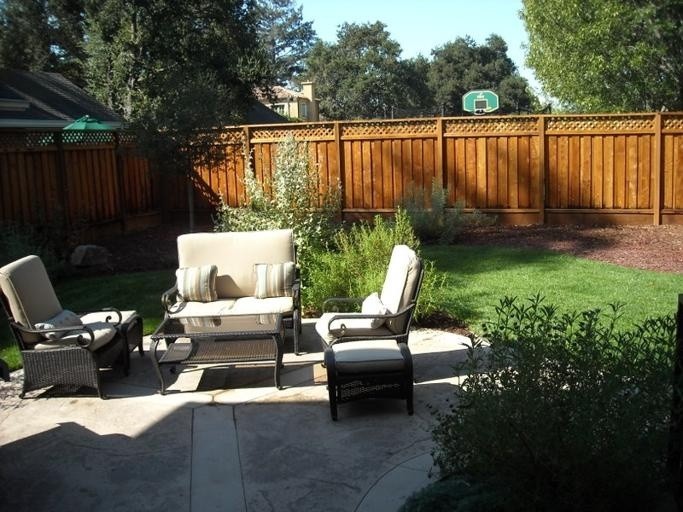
324,340,414,422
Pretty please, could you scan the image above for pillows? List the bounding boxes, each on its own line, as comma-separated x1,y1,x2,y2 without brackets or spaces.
254,314,278,326
179,317,222,327
0,253,83,346
360,245,421,329
173,230,297,298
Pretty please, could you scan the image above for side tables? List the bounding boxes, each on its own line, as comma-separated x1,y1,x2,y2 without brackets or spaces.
80,309,144,376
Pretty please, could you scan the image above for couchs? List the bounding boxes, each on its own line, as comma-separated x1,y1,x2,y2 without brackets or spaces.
160,229,302,355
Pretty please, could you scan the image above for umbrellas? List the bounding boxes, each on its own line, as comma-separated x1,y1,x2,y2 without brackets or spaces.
38,111,132,146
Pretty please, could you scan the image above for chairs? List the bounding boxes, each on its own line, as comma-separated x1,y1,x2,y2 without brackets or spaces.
314,244,425,341
0,254,129,400
